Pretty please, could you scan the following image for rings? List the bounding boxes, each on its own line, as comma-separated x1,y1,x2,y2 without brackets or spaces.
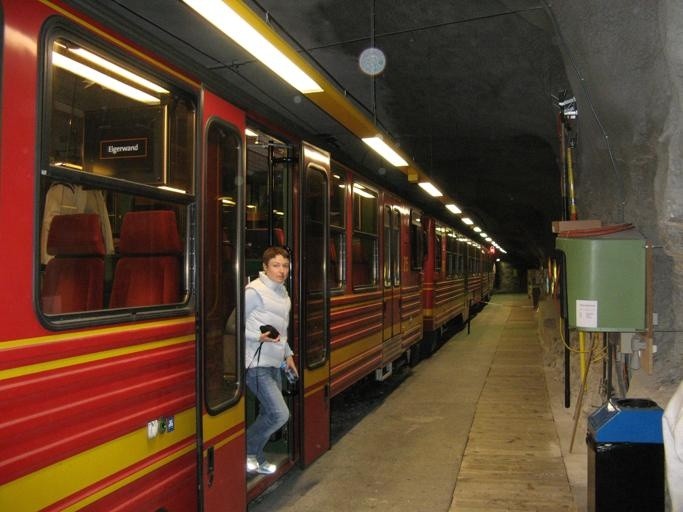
272,339,275,342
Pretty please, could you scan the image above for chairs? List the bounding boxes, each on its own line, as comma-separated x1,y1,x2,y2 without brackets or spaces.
40,211,186,315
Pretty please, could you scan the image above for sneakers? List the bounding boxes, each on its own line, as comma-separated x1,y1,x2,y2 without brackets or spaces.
247,455,277,474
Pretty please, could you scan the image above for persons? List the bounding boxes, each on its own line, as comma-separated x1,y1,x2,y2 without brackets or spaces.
227,246,300,474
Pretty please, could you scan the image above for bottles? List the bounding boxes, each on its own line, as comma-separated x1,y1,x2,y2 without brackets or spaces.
280,359,298,386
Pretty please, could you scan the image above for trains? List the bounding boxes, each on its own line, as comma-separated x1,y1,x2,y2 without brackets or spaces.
0,1,499,512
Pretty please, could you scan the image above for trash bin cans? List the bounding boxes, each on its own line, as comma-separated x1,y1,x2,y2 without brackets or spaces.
586,397,665,512
